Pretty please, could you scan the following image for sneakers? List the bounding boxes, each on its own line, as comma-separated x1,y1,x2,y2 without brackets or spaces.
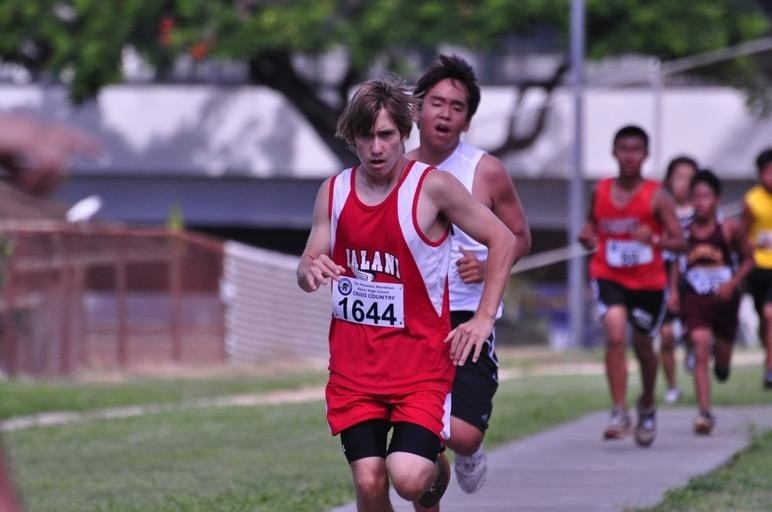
694,409,714,436
454,449,488,494
666,387,684,403
417,452,450,508
634,393,657,446
603,407,631,437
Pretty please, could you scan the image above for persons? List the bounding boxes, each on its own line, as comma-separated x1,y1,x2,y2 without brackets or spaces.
741,147,772,393
576,121,689,448
405,52,532,496
660,154,700,405
665,167,757,434
293,75,521,510
